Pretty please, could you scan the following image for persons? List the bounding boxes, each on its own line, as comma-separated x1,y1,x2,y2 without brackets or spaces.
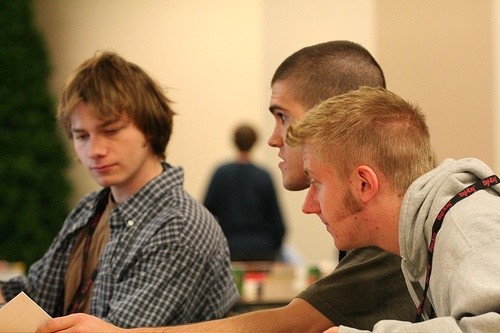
33,41,424,333
0,49,241,328
202,125,287,262
284,84,500,333
